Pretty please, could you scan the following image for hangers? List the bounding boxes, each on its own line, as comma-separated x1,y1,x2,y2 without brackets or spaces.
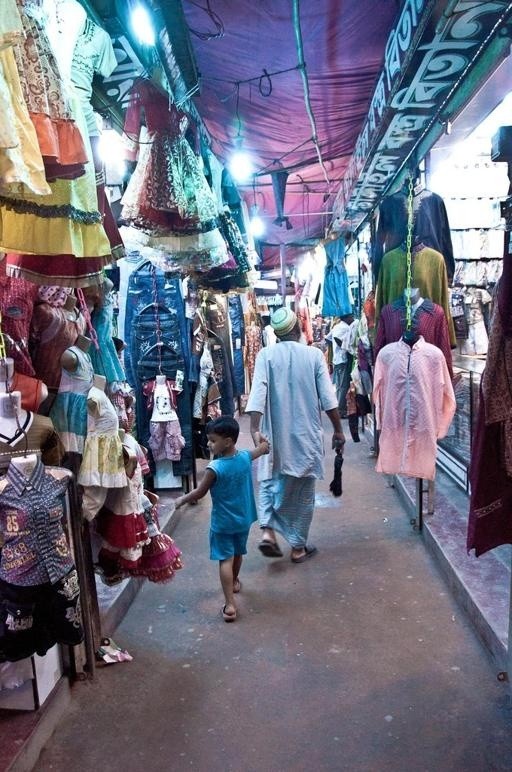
131,256,182,361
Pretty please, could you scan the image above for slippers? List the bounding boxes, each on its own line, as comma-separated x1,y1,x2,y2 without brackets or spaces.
291,543,316,563
257,540,283,558
221,601,236,621
234,578,241,593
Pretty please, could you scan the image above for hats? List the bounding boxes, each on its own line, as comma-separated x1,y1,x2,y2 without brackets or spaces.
270,307,297,336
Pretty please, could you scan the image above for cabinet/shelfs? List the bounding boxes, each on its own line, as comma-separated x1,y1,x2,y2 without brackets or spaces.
436,352,487,495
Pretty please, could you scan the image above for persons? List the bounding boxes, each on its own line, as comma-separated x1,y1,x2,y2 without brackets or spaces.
125,63,217,229
54,334,94,452
458,286,492,359
0,388,54,468
249,308,347,564
88,275,127,383
96,419,178,580
2,357,48,412
34,297,86,396
375,233,450,327
1,455,82,664
371,175,455,290
43,1,120,139
173,414,272,624
144,374,184,462
325,308,354,422
315,228,355,320
376,286,453,379
369,331,452,482
79,374,124,521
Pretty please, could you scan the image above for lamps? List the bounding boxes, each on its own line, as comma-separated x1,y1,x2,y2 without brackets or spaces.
227,83,252,182
248,178,265,237
